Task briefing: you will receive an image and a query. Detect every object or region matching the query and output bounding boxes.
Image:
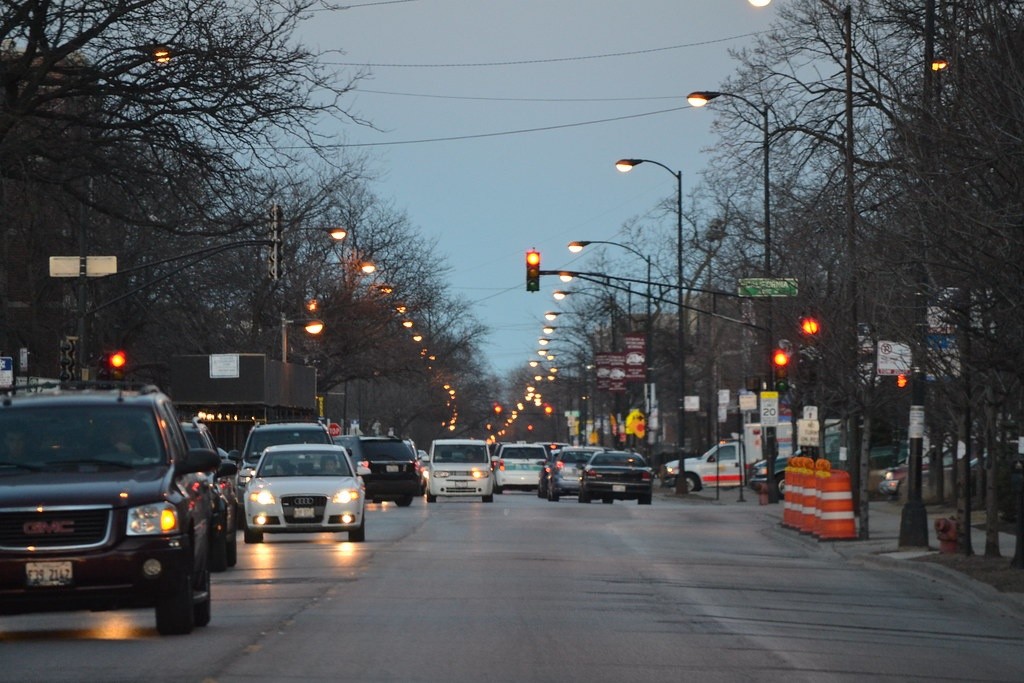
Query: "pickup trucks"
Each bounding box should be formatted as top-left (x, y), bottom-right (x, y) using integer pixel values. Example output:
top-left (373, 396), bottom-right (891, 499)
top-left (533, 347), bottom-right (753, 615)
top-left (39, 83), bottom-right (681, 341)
top-left (749, 432), bottom-right (897, 504)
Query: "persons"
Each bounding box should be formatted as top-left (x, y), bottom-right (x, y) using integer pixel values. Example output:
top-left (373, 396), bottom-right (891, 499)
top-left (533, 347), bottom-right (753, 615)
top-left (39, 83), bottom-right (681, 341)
top-left (324, 457), bottom-right (336, 470)
top-left (466, 449), bottom-right (474, 460)
top-left (109, 418), bottom-right (136, 453)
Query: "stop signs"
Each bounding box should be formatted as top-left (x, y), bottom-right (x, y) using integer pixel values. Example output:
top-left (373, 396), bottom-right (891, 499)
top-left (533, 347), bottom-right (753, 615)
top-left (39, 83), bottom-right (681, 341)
top-left (329, 423), bottom-right (342, 436)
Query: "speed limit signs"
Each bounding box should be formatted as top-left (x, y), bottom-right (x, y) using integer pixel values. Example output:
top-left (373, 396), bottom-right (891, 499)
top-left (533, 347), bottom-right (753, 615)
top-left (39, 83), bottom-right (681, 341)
top-left (759, 392), bottom-right (779, 428)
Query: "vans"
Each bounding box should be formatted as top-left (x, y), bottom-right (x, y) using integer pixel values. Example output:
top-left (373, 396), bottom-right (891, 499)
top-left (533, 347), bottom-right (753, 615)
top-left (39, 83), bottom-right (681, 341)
top-left (491, 444), bottom-right (548, 496)
top-left (421, 438), bottom-right (499, 503)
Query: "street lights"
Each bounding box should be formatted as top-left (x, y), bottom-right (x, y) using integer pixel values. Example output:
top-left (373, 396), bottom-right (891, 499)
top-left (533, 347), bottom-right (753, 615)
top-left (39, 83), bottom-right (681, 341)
top-left (568, 239), bottom-right (652, 468)
top-left (616, 157), bottom-right (688, 495)
top-left (687, 91), bottom-right (776, 507)
top-left (748, 0), bottom-right (860, 523)
top-left (282, 312), bottom-right (324, 363)
top-left (530, 272), bottom-right (637, 450)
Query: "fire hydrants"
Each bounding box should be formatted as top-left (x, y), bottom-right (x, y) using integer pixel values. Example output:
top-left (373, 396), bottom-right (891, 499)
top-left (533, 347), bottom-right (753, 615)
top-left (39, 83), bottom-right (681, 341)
top-left (934, 516), bottom-right (959, 555)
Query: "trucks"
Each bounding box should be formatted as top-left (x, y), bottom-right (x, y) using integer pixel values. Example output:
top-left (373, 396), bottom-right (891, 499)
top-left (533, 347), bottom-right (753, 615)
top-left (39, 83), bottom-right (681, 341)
top-left (660, 416), bottom-right (842, 496)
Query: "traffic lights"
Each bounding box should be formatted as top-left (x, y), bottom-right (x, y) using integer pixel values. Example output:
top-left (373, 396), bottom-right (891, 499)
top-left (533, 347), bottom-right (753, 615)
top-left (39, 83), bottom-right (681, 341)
top-left (526, 252), bottom-right (541, 291)
top-left (773, 353), bottom-right (788, 395)
top-left (108, 351), bottom-right (127, 388)
top-left (896, 374), bottom-right (909, 388)
top-left (96, 356), bottom-right (111, 390)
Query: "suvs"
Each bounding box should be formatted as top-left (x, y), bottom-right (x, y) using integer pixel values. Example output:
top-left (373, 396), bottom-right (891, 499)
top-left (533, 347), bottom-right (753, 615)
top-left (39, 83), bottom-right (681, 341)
top-left (330, 434), bottom-right (425, 507)
top-left (227, 421), bottom-right (353, 530)
top-left (0, 381), bottom-right (223, 637)
top-left (179, 416), bottom-right (240, 572)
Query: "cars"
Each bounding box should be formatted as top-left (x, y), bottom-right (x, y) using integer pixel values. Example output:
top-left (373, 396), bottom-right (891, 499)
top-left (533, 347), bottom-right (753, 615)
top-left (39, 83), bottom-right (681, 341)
top-left (403, 438), bottom-right (432, 482)
top-left (491, 439), bottom-right (571, 456)
top-left (878, 447), bottom-right (989, 506)
top-left (576, 449), bottom-right (654, 505)
top-left (238, 443), bottom-right (373, 544)
top-left (536, 446), bottom-right (605, 502)
